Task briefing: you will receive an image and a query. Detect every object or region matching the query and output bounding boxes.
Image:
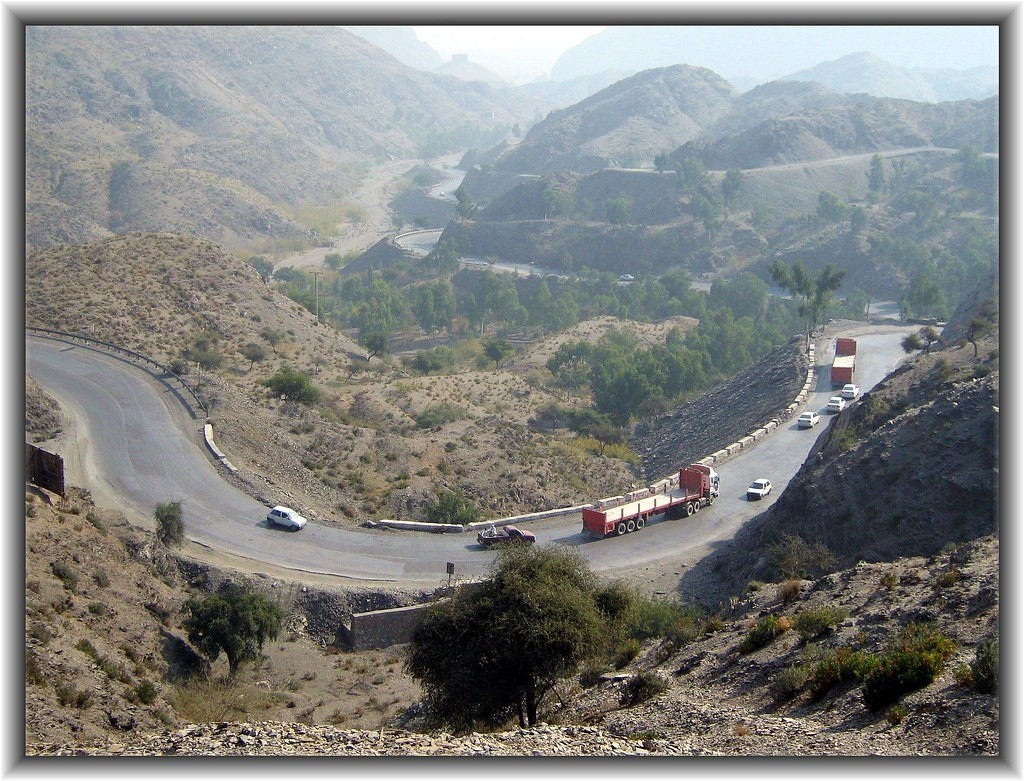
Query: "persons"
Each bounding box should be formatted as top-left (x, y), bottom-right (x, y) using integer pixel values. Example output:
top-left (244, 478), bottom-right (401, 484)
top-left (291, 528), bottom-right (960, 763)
top-left (483, 528), bottom-right (493, 537)
top-left (489, 525), bottom-right (497, 536)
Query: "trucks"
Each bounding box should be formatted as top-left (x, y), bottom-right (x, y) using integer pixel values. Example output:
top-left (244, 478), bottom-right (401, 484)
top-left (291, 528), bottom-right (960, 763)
top-left (829, 337), bottom-right (857, 388)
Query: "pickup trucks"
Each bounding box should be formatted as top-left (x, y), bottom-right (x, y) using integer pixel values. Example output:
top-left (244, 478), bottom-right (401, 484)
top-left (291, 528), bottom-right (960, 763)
top-left (476, 524), bottom-right (536, 551)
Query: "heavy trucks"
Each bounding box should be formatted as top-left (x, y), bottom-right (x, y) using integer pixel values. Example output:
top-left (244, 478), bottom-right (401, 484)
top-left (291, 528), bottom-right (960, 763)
top-left (581, 462), bottom-right (721, 541)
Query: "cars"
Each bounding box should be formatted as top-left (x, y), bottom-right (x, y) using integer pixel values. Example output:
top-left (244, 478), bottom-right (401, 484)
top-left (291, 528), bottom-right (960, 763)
top-left (841, 383), bottom-right (860, 400)
top-left (826, 396), bottom-right (847, 414)
top-left (746, 478), bottom-right (772, 500)
top-left (619, 274), bottom-right (635, 281)
top-left (266, 505), bottom-right (308, 532)
top-left (797, 411), bottom-right (821, 429)
